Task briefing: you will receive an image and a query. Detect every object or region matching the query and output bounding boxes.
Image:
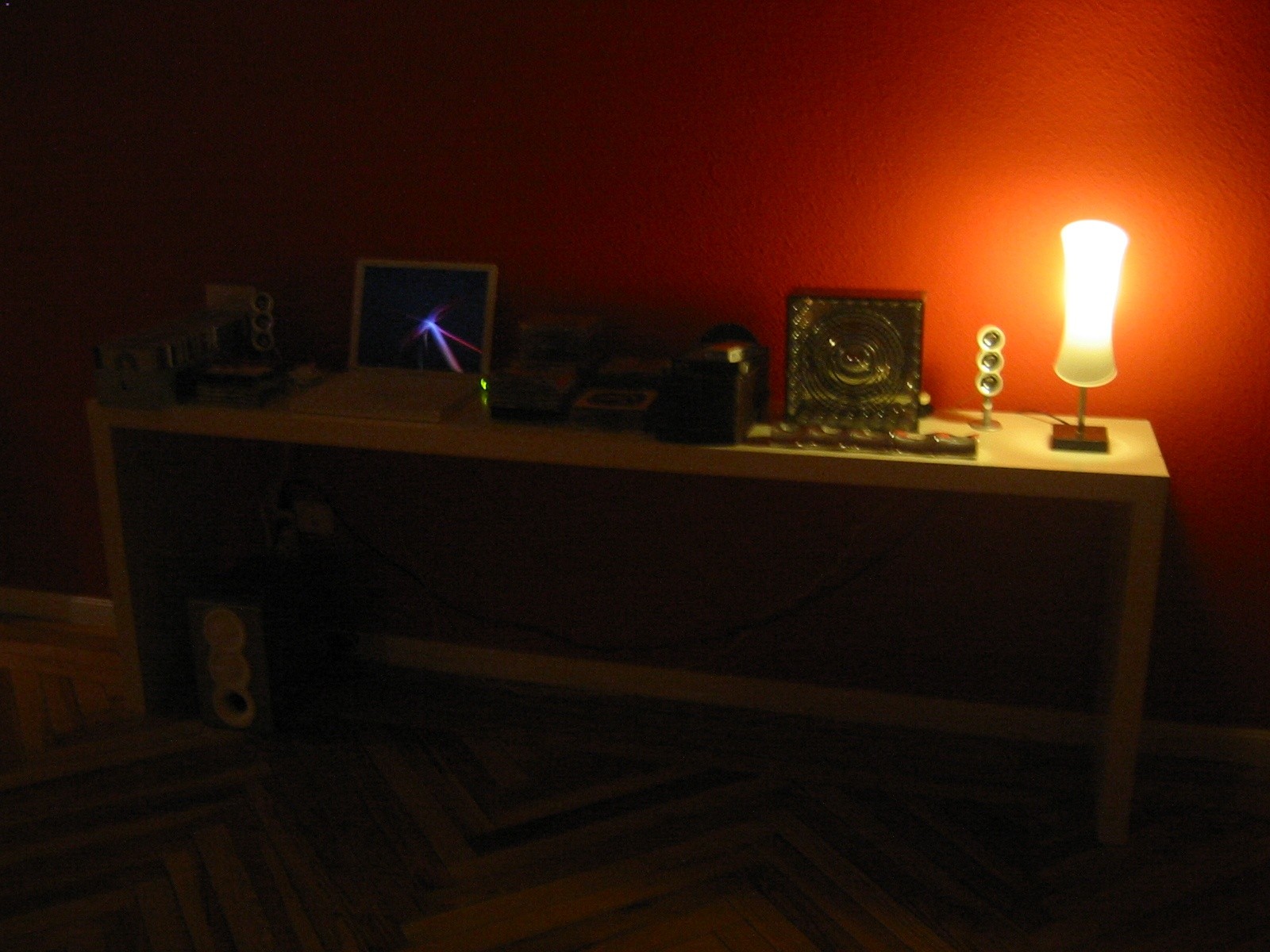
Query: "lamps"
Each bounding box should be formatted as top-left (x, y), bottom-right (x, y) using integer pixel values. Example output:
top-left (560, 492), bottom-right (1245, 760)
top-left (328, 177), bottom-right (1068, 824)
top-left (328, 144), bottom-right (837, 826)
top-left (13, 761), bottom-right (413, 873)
top-left (1051, 219), bottom-right (1128, 452)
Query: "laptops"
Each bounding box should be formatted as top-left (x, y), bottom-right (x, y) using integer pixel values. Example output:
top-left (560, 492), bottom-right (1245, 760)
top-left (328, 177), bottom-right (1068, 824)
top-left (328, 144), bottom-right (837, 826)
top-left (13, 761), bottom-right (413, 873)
top-left (288, 260), bottom-right (497, 424)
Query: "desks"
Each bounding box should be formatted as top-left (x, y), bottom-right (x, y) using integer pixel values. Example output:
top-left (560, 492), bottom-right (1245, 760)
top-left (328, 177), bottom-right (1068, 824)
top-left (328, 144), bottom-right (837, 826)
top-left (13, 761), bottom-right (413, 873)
top-left (84, 358), bottom-right (1170, 842)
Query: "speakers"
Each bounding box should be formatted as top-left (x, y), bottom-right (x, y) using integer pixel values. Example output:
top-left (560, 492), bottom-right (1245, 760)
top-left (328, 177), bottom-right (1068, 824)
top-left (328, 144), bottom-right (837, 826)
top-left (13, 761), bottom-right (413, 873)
top-left (973, 324), bottom-right (1007, 428)
top-left (250, 292), bottom-right (277, 355)
top-left (188, 596), bottom-right (282, 737)
